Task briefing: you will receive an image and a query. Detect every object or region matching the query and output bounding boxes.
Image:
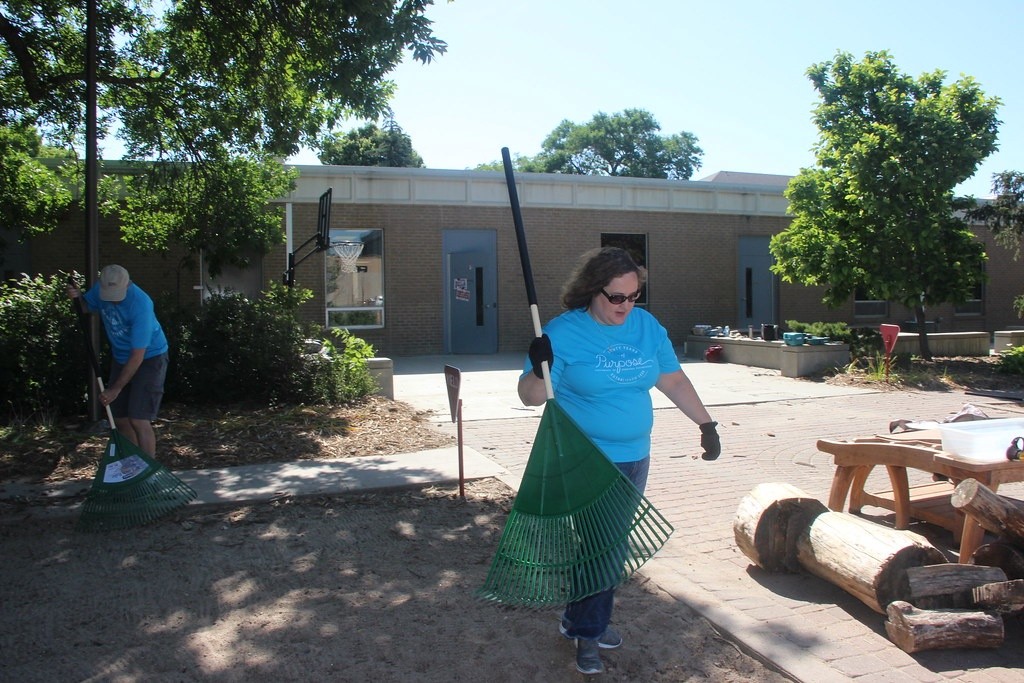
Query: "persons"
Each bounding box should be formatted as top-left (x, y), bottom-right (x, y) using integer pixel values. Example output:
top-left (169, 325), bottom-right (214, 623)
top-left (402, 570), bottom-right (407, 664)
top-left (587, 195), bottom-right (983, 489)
top-left (67, 264), bottom-right (168, 495)
top-left (528, 248), bottom-right (720, 676)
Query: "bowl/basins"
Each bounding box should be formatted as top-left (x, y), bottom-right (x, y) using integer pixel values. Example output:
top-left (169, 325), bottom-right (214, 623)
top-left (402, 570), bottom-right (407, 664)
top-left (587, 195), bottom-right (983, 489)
top-left (691, 328), bottom-right (710, 336)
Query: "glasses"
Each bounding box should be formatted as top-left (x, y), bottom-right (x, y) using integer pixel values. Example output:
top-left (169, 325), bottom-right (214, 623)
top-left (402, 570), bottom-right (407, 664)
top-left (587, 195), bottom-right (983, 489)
top-left (600, 287), bottom-right (641, 305)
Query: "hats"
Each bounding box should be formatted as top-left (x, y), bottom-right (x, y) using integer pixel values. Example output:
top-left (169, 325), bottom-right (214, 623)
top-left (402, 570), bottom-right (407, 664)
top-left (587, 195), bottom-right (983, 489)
top-left (99, 264), bottom-right (129, 301)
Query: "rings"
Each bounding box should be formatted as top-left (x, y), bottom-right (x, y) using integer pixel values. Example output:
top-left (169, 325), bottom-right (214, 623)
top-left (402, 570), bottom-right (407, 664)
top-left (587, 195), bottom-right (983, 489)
top-left (104, 400), bottom-right (107, 402)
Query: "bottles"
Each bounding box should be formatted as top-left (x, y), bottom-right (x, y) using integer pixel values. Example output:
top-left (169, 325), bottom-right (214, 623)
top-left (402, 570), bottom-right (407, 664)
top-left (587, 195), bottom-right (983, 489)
top-left (725, 325), bottom-right (729, 337)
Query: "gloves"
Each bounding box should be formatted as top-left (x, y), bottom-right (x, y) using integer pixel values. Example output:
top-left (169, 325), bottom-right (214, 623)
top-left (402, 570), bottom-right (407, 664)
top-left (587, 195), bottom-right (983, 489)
top-left (699, 422), bottom-right (720, 460)
top-left (528, 334), bottom-right (554, 379)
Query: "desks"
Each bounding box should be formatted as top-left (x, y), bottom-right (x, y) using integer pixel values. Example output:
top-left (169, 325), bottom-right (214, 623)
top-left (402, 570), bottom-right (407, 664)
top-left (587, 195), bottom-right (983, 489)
top-left (817, 415), bottom-right (1024, 565)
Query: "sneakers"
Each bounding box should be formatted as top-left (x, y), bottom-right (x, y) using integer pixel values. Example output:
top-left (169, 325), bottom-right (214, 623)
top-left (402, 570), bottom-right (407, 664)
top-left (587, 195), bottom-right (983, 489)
top-left (559, 620), bottom-right (622, 648)
top-left (572, 637), bottom-right (603, 674)
